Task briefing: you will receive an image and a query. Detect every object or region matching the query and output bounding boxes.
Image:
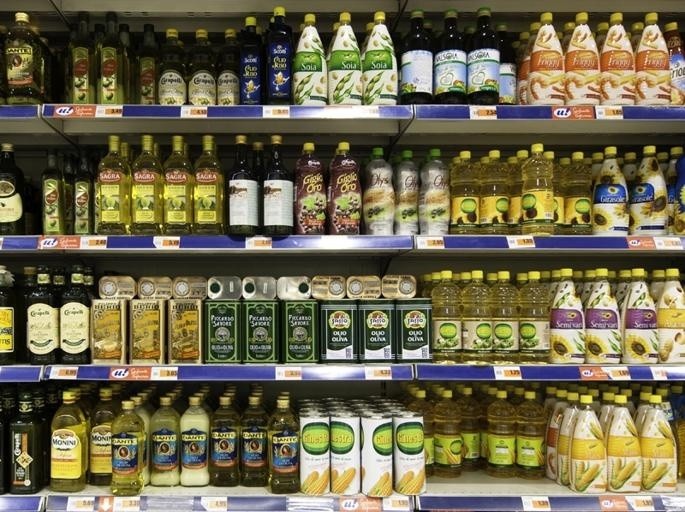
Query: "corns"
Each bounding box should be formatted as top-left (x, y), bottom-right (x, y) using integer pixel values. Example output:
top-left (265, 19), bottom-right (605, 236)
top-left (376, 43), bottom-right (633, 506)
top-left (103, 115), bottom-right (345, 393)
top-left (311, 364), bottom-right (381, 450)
top-left (561, 459), bottom-right (668, 491)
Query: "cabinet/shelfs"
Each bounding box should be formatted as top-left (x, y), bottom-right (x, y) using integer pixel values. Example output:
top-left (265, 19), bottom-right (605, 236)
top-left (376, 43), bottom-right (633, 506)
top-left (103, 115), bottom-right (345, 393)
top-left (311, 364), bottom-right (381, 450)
top-left (0, 0), bottom-right (685, 512)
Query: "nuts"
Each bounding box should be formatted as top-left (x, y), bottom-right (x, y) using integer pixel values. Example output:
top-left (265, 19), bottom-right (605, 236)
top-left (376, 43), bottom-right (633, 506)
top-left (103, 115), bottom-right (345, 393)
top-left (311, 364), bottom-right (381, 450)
top-left (660, 339), bottom-right (675, 361)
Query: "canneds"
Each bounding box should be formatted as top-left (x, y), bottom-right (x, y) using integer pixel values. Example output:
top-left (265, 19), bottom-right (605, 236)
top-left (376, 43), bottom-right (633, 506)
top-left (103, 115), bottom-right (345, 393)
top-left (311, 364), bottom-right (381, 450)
top-left (298, 396), bottom-right (427, 497)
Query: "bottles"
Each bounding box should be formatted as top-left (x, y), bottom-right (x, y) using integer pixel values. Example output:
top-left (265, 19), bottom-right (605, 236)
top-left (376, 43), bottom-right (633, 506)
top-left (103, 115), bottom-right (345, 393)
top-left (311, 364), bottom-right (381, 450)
top-left (0, 383), bottom-right (685, 496)
top-left (0, 261), bottom-right (685, 364)
top-left (3, 8), bottom-right (685, 107)
top-left (4, 133), bottom-right (685, 235)
